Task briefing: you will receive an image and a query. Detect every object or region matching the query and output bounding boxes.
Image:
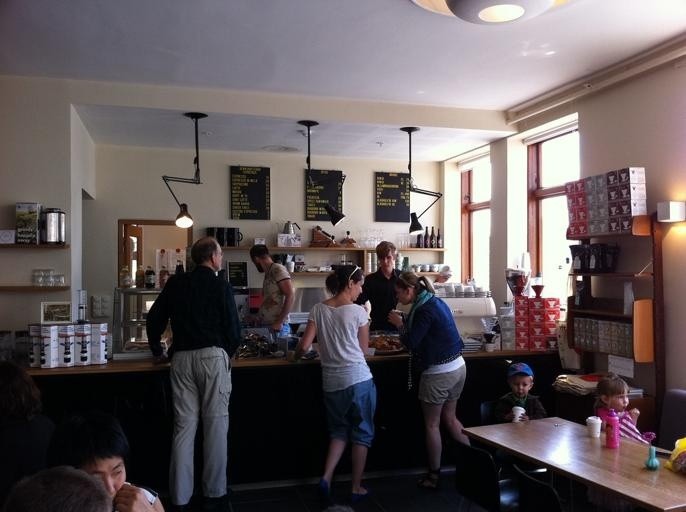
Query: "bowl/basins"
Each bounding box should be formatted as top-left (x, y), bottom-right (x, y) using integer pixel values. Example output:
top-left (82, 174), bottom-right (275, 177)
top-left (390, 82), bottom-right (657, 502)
top-left (410, 263), bottom-right (446, 272)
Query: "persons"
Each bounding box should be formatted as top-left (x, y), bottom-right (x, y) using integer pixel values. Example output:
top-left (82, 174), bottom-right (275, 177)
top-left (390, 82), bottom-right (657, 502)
top-left (389, 270), bottom-right (472, 491)
top-left (355, 240), bottom-right (405, 330)
top-left (5, 462), bottom-right (112, 510)
top-left (47, 412), bottom-right (166, 511)
top-left (146, 236), bottom-right (241, 509)
top-left (592, 374), bottom-right (651, 446)
top-left (243, 245), bottom-right (293, 338)
top-left (294, 263), bottom-right (382, 500)
top-left (495, 363), bottom-right (546, 423)
top-left (1, 359), bottom-right (58, 509)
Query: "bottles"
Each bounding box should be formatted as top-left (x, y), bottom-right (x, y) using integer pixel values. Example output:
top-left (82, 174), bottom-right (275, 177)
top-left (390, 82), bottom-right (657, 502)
top-left (645, 447), bottom-right (660, 471)
top-left (623, 281), bottom-right (634, 316)
top-left (603, 408), bottom-right (621, 449)
top-left (417, 226), bottom-right (443, 248)
top-left (118, 259), bottom-right (184, 289)
top-left (40, 206), bottom-right (66, 244)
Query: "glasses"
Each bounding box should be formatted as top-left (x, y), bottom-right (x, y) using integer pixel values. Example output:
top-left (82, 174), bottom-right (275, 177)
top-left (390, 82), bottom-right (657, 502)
top-left (348, 262), bottom-right (362, 281)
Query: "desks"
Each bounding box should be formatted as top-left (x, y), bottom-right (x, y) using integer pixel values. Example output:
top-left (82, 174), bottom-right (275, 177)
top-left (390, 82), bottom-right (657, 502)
top-left (457, 413), bottom-right (686, 510)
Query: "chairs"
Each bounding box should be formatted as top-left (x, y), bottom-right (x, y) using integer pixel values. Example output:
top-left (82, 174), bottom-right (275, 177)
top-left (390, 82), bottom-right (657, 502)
top-left (451, 440), bottom-right (524, 510)
top-left (513, 459), bottom-right (566, 510)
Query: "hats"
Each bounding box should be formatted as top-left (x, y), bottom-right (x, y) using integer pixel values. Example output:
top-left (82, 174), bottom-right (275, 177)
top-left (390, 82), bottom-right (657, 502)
top-left (506, 362), bottom-right (535, 380)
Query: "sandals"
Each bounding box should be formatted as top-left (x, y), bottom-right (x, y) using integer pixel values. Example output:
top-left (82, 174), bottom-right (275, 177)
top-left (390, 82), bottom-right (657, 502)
top-left (415, 467), bottom-right (442, 492)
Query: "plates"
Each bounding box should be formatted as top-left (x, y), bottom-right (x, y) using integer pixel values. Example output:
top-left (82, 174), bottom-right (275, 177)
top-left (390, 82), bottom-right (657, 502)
top-left (458, 339), bottom-right (481, 353)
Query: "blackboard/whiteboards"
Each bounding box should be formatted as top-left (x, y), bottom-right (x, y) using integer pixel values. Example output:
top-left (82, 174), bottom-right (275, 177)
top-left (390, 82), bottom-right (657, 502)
top-left (230, 166), bottom-right (271, 221)
top-left (226, 260), bottom-right (249, 288)
top-left (374, 172), bottom-right (411, 222)
top-left (305, 168), bottom-right (342, 221)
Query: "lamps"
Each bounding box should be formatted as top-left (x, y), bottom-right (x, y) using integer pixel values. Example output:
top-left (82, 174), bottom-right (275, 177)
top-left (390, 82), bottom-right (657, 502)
top-left (293, 120), bottom-right (346, 228)
top-left (407, 1), bottom-right (578, 31)
top-left (158, 112), bottom-right (210, 230)
top-left (398, 125), bottom-right (444, 237)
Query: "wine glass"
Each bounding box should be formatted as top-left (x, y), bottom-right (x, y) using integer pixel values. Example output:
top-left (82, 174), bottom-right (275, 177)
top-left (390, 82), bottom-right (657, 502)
top-left (531, 284), bottom-right (544, 299)
top-left (567, 171), bottom-right (632, 236)
top-left (518, 300), bottom-right (559, 349)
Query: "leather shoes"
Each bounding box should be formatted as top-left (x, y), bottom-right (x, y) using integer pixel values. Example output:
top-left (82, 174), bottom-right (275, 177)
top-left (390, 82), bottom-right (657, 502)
top-left (173, 487), bottom-right (199, 512)
top-left (347, 483), bottom-right (374, 505)
top-left (200, 486), bottom-right (234, 512)
top-left (318, 477), bottom-right (336, 507)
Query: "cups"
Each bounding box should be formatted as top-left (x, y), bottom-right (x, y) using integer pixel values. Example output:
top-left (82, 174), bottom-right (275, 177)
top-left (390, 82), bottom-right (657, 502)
top-left (288, 262), bottom-right (294, 273)
top-left (512, 406), bottom-right (527, 423)
top-left (29, 267), bottom-right (66, 285)
top-left (505, 267), bottom-right (531, 296)
top-left (500, 306), bottom-right (514, 315)
top-left (366, 251), bottom-right (409, 273)
top-left (392, 310), bottom-right (404, 317)
top-left (482, 317), bottom-right (502, 352)
top-left (433, 283), bottom-right (494, 298)
top-left (586, 415), bottom-right (602, 438)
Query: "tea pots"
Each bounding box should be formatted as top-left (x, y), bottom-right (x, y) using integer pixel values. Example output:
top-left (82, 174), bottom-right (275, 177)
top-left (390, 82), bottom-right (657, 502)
top-left (283, 220), bottom-right (301, 234)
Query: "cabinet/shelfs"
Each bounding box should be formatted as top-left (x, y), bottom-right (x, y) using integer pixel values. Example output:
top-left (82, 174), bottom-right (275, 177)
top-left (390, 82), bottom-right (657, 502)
top-left (0, 240), bottom-right (70, 292)
top-left (557, 206), bottom-right (667, 438)
top-left (192, 241), bottom-right (448, 283)
